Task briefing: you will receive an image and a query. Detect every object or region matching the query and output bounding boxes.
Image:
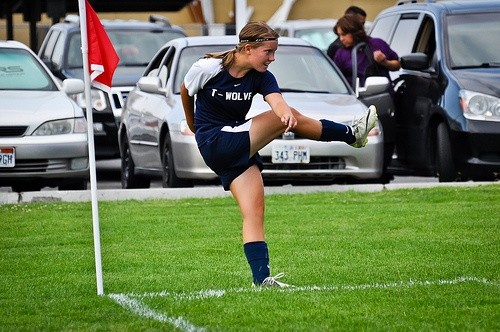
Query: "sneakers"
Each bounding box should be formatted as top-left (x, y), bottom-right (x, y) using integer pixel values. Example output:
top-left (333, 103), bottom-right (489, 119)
top-left (252, 277), bottom-right (289, 288)
top-left (350, 104), bottom-right (378, 148)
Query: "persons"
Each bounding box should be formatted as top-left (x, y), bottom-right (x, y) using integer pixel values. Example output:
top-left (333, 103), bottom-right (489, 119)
top-left (181, 21), bottom-right (379, 288)
top-left (328, 6), bottom-right (400, 185)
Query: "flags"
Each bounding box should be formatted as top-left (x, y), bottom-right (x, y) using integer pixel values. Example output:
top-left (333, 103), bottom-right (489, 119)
top-left (79, 0), bottom-right (120, 93)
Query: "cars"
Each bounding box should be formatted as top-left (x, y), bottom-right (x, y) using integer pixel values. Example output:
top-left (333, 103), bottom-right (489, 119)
top-left (118, 35), bottom-right (385, 189)
top-left (0, 40), bottom-right (90, 192)
top-left (272, 20), bottom-right (374, 55)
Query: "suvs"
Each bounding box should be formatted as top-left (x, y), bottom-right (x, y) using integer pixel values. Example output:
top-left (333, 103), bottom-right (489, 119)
top-left (37, 14), bottom-right (188, 136)
top-left (369, 0), bottom-right (500, 180)
